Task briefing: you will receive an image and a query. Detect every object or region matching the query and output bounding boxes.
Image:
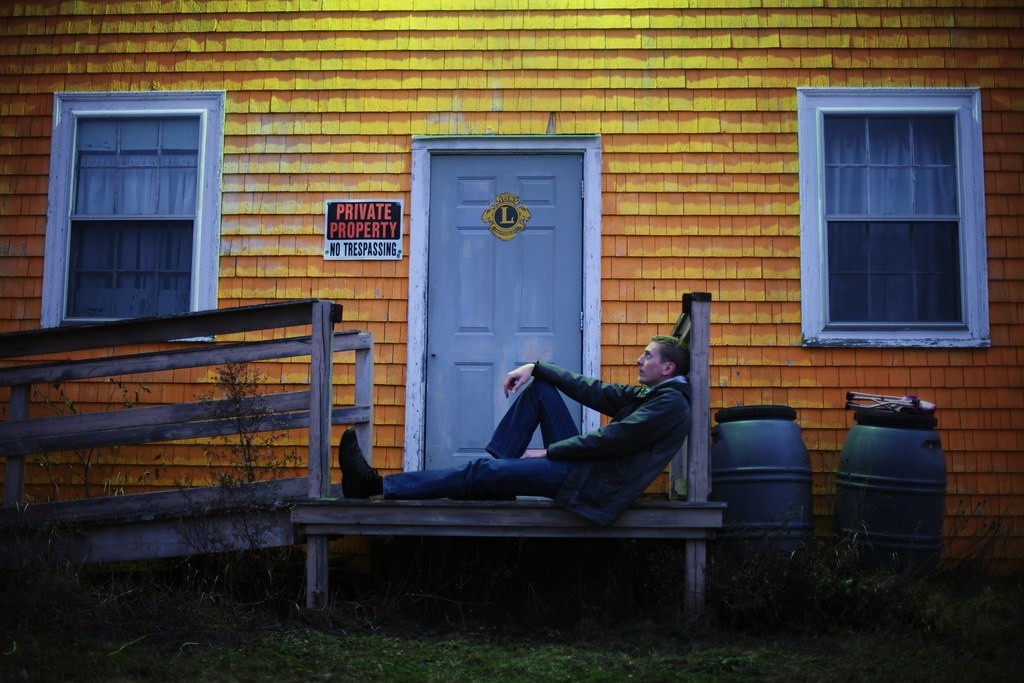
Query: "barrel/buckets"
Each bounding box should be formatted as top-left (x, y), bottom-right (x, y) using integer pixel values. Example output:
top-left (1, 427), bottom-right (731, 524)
top-left (702, 418), bottom-right (816, 602)
top-left (831, 424), bottom-right (947, 581)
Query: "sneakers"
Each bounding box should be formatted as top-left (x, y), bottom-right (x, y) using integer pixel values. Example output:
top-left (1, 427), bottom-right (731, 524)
top-left (339, 427), bottom-right (379, 499)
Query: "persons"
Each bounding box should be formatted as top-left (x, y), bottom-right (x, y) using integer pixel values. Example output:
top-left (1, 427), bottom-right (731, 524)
top-left (336, 334), bottom-right (689, 527)
